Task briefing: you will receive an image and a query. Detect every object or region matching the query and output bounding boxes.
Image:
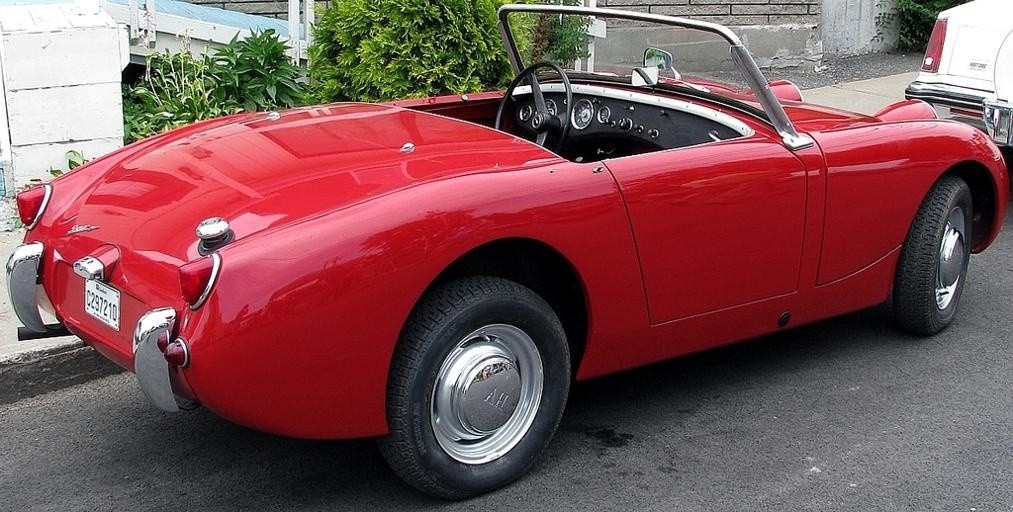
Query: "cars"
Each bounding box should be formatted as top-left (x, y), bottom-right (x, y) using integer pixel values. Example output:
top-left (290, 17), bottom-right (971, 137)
top-left (903, 2), bottom-right (1013, 157)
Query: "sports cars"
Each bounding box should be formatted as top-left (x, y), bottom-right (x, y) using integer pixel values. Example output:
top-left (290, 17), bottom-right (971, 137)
top-left (5, 5), bottom-right (1008, 502)
